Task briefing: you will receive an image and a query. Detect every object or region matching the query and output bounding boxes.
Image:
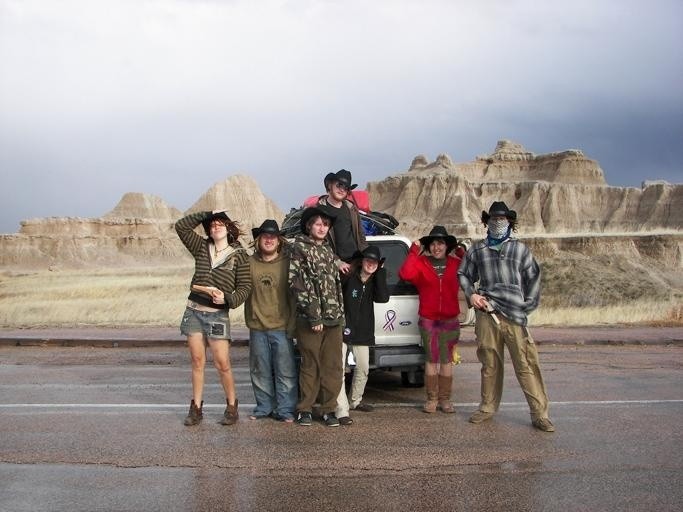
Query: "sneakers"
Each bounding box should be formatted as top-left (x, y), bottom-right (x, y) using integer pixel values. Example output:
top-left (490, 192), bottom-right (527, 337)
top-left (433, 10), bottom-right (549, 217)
top-left (532, 417), bottom-right (555, 432)
top-left (338, 417), bottom-right (353, 426)
top-left (297, 412), bottom-right (313, 426)
top-left (320, 411), bottom-right (340, 427)
top-left (350, 403), bottom-right (375, 412)
top-left (469, 409), bottom-right (495, 424)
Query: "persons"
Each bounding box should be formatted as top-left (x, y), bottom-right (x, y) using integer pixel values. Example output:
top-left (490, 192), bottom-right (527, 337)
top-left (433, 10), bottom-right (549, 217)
top-left (398, 225), bottom-right (468, 413)
top-left (175, 209), bottom-right (253, 427)
top-left (456, 200), bottom-right (556, 432)
top-left (304, 168), bottom-right (370, 281)
top-left (288, 206), bottom-right (346, 427)
top-left (245, 219), bottom-right (303, 423)
top-left (335, 245), bottom-right (389, 425)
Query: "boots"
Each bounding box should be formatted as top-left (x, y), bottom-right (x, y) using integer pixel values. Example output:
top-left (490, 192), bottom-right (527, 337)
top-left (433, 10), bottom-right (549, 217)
top-left (220, 397), bottom-right (240, 426)
top-left (423, 373), bottom-right (456, 414)
top-left (183, 399), bottom-right (204, 427)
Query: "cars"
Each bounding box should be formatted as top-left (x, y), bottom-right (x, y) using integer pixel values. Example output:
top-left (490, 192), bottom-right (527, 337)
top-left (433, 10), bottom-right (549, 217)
top-left (281, 234), bottom-right (473, 389)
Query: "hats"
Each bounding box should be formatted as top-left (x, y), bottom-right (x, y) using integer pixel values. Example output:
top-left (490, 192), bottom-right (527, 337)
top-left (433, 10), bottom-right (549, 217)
top-left (251, 219), bottom-right (287, 241)
top-left (300, 206), bottom-right (338, 237)
top-left (202, 212), bottom-right (239, 243)
top-left (419, 225), bottom-right (458, 256)
top-left (352, 245), bottom-right (386, 271)
top-left (324, 169), bottom-right (358, 191)
top-left (481, 200), bottom-right (517, 224)
